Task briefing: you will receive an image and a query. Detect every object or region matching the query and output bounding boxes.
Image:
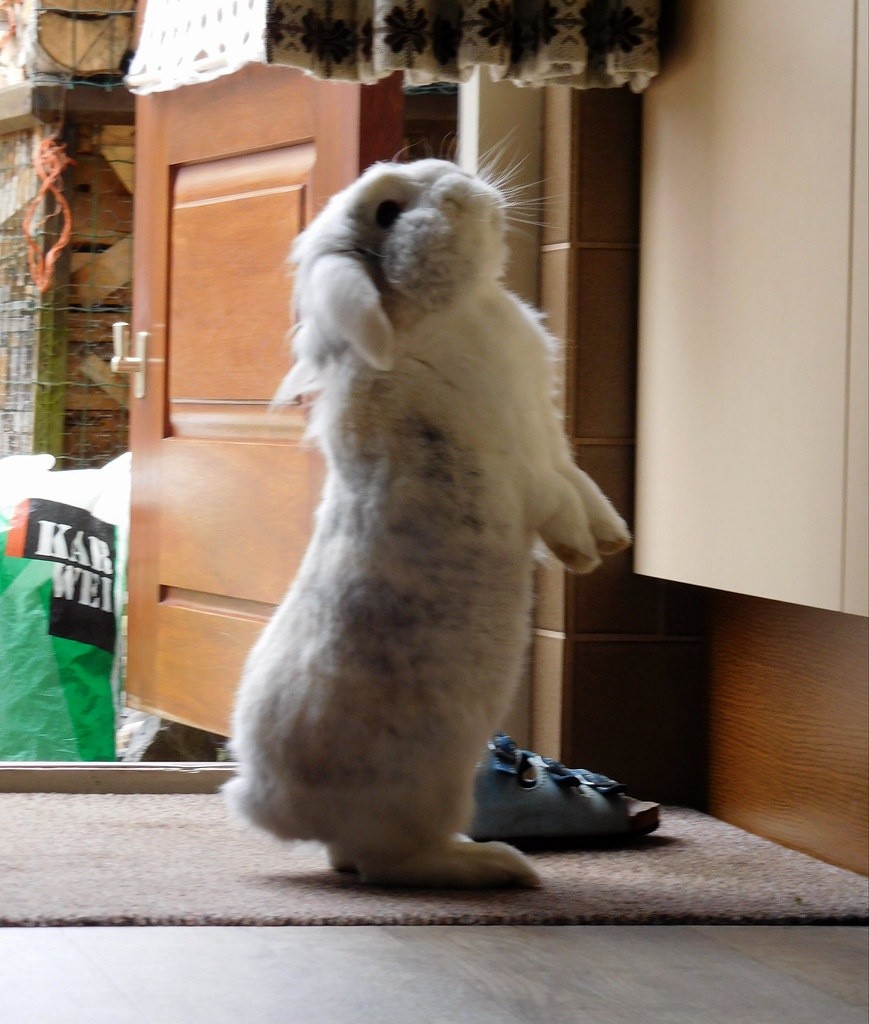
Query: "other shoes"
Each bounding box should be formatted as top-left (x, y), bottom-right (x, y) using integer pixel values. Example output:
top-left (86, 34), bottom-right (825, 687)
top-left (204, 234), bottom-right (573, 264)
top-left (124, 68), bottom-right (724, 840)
top-left (463, 733), bottom-right (662, 847)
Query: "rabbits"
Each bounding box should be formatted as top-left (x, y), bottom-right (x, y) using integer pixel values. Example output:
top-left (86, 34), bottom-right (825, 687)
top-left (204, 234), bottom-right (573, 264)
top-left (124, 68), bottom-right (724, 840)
top-left (212, 128), bottom-right (630, 888)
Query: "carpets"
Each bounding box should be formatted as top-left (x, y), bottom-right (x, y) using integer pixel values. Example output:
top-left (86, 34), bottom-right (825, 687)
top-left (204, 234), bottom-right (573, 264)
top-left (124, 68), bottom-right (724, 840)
top-left (0, 791), bottom-right (869, 929)
